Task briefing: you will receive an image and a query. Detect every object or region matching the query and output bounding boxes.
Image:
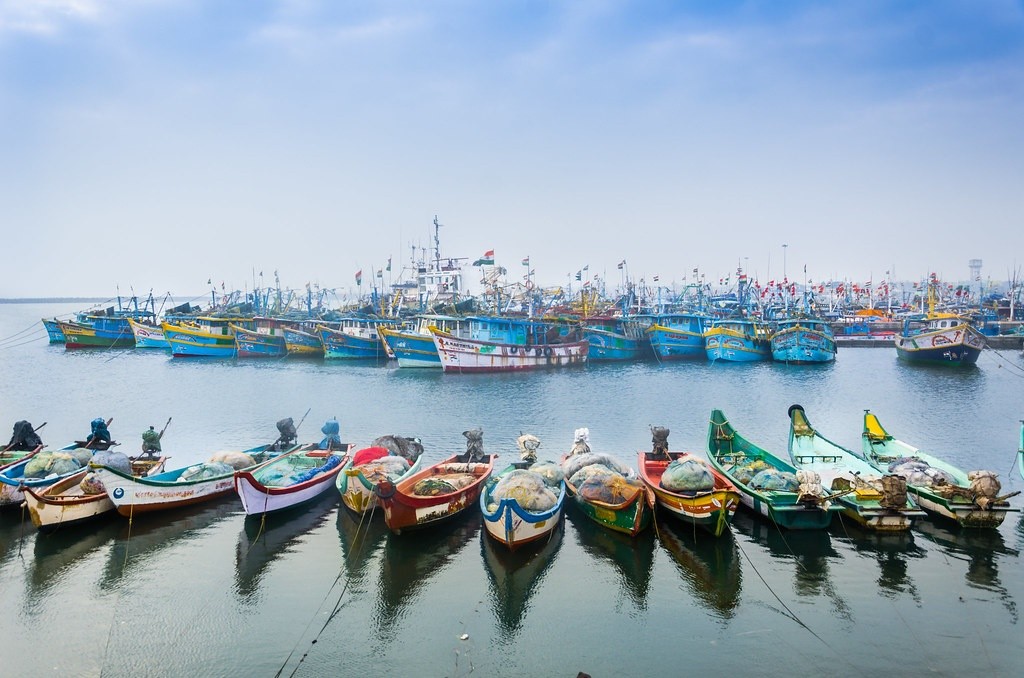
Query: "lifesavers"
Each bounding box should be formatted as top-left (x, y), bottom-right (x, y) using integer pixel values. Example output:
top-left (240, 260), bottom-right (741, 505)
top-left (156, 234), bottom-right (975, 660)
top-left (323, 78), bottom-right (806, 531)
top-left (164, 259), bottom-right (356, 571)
top-left (536, 349), bottom-right (542, 355)
top-left (753, 338), bottom-right (759, 346)
top-left (760, 339), bottom-right (769, 345)
top-left (544, 347), bottom-right (552, 356)
top-left (511, 346), bottom-right (517, 353)
top-left (951, 308), bottom-right (959, 315)
top-left (525, 347), bottom-right (531, 351)
top-left (637, 339), bottom-right (641, 346)
top-left (375, 481), bottom-right (396, 498)
top-left (745, 335), bottom-right (750, 340)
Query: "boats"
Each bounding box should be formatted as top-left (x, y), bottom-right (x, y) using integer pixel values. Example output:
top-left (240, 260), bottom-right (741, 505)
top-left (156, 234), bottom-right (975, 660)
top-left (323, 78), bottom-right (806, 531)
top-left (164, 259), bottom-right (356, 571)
top-left (336, 434), bottom-right (425, 515)
top-left (0, 416), bottom-right (169, 530)
top-left (43, 212), bottom-right (1024, 373)
top-left (637, 425), bottom-right (742, 528)
top-left (87, 416), bottom-right (303, 522)
top-left (1018, 422), bottom-right (1024, 478)
top-left (376, 428), bottom-right (499, 533)
top-left (706, 408), bottom-right (848, 531)
top-left (787, 404), bottom-right (928, 531)
top-left (562, 428), bottom-right (646, 536)
top-left (862, 409), bottom-right (1011, 527)
top-left (480, 433), bottom-right (566, 550)
top-left (234, 419), bottom-right (355, 517)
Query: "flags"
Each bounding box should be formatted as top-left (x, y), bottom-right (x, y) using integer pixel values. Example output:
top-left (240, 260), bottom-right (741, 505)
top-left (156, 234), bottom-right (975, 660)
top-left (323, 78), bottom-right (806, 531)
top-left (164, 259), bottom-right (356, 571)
top-left (693, 268), bottom-right (705, 279)
top-left (653, 275), bottom-right (658, 281)
top-left (472, 250), bottom-right (495, 266)
top-left (804, 265), bottom-right (904, 306)
top-left (912, 273), bottom-right (969, 299)
top-left (975, 275), bottom-right (998, 294)
top-left (761, 277), bottom-right (795, 301)
top-left (306, 282), bottom-right (319, 292)
top-left (442, 276), bottom-right (454, 289)
top-left (355, 271), bottom-right (362, 286)
top-left (682, 276), bottom-right (687, 279)
top-left (258, 270), bottom-right (280, 282)
top-left (719, 267), bottom-right (760, 289)
top-left (208, 279), bottom-right (226, 292)
top-left (117, 285), bottom-right (154, 292)
top-left (522, 259), bottom-right (535, 280)
top-left (377, 258), bottom-right (391, 277)
top-left (617, 259), bottom-right (626, 270)
top-left (640, 278), bottom-right (644, 283)
top-left (566, 264), bottom-right (598, 288)
